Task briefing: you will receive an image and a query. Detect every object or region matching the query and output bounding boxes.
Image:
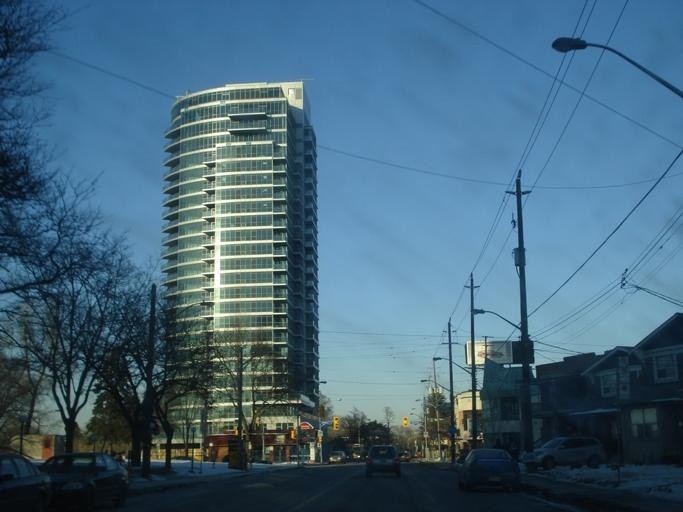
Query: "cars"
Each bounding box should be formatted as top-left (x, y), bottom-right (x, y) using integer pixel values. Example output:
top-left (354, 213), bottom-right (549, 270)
top-left (0, 453), bottom-right (51, 511)
top-left (38, 452), bottom-right (128, 511)
top-left (363, 444), bottom-right (402, 478)
top-left (327, 443), bottom-right (411, 465)
top-left (457, 447), bottom-right (521, 492)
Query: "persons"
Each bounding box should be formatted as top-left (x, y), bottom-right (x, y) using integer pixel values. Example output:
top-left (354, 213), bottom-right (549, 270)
top-left (460, 444), bottom-right (469, 459)
top-left (493, 438), bottom-right (505, 449)
top-left (346, 455), bottom-right (350, 462)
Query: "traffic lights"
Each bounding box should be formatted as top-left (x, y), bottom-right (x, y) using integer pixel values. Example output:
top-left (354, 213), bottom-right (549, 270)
top-left (402, 415), bottom-right (408, 426)
top-left (333, 416), bottom-right (339, 431)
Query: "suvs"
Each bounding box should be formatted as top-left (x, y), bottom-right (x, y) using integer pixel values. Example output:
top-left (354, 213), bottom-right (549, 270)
top-left (521, 435), bottom-right (606, 471)
top-left (538, 434), bottom-right (613, 459)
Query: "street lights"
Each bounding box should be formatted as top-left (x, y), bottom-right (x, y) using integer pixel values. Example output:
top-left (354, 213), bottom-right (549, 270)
top-left (408, 398), bottom-right (442, 460)
top-left (296, 380), bottom-right (327, 464)
top-left (189, 425), bottom-right (196, 471)
top-left (18, 413), bottom-right (28, 457)
top-left (420, 379), bottom-right (455, 464)
top-left (140, 300), bottom-right (214, 478)
top-left (551, 37), bottom-right (682, 99)
top-left (432, 357), bottom-right (476, 450)
top-left (319, 398), bottom-right (341, 463)
top-left (470, 308), bottom-right (535, 474)
